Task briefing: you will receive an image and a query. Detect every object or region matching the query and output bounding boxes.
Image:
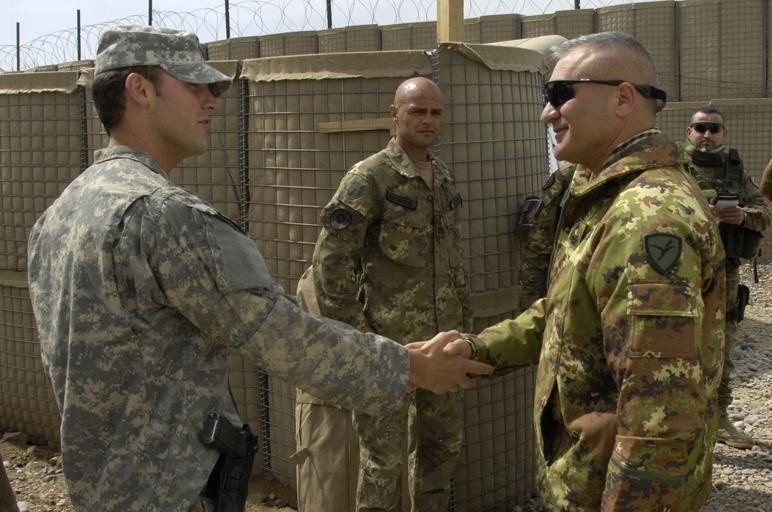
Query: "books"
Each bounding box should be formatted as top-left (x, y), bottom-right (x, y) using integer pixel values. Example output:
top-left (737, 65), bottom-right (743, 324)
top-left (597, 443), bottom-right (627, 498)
top-left (712, 193), bottom-right (741, 223)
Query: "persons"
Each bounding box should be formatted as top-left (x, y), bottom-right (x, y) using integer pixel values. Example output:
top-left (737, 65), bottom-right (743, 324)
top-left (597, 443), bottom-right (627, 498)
top-left (673, 106), bottom-right (772, 450)
top-left (310, 76), bottom-right (478, 511)
top-left (25, 25), bottom-right (496, 512)
top-left (401, 31), bottom-right (727, 512)
top-left (520, 161), bottom-right (577, 312)
top-left (759, 158), bottom-right (772, 201)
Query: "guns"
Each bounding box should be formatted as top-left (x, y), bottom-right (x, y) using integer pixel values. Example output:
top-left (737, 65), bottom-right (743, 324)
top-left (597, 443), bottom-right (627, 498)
top-left (195, 413), bottom-right (258, 511)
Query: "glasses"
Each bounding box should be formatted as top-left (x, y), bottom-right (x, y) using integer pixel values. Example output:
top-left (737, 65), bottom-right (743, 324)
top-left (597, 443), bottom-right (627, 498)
top-left (688, 120), bottom-right (727, 135)
top-left (541, 75), bottom-right (642, 109)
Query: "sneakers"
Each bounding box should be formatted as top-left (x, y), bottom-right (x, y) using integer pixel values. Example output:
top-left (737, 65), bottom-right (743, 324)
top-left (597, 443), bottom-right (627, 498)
top-left (715, 405), bottom-right (754, 449)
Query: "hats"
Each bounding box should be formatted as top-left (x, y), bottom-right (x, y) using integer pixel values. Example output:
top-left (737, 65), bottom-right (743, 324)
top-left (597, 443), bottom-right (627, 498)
top-left (93, 25), bottom-right (233, 99)
